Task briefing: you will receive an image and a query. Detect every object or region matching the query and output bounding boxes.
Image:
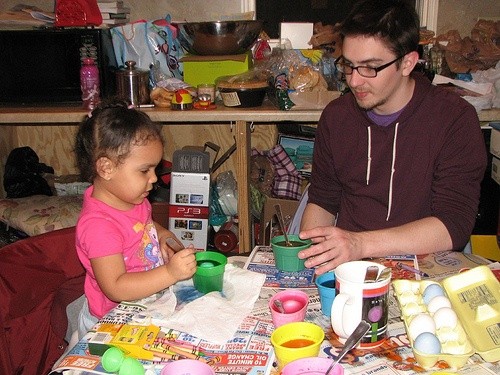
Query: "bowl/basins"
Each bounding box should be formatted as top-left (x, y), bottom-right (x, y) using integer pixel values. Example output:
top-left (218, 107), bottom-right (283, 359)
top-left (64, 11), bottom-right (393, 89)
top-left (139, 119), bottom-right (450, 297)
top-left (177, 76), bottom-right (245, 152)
top-left (170, 20), bottom-right (263, 56)
top-left (217, 86), bottom-right (270, 108)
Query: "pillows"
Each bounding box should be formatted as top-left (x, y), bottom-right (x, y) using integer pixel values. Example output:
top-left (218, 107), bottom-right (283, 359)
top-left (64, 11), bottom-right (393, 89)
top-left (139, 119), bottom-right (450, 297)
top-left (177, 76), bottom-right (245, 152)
top-left (0, 195), bottom-right (80, 237)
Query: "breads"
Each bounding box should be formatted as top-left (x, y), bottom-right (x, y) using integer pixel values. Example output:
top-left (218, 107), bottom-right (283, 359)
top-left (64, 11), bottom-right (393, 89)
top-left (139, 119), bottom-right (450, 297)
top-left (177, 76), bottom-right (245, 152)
top-left (289, 69), bottom-right (328, 90)
top-left (149, 85), bottom-right (176, 108)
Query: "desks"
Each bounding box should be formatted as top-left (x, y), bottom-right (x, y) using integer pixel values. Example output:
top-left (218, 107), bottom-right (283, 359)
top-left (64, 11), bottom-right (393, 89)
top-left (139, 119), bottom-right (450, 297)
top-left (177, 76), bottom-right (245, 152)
top-left (48, 251), bottom-right (492, 374)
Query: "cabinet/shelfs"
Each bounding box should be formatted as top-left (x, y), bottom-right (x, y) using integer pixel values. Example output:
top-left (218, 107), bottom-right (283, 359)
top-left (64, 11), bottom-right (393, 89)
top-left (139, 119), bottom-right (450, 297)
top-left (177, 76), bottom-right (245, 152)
top-left (0, 107), bottom-right (500, 255)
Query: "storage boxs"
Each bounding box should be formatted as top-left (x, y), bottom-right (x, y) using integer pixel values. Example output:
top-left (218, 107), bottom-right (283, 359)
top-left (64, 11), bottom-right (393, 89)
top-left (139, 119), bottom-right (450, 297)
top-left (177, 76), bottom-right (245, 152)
top-left (0, 29), bottom-right (117, 107)
top-left (183, 50), bottom-right (253, 87)
top-left (261, 196), bottom-right (298, 246)
top-left (274, 123), bottom-right (316, 176)
top-left (167, 146), bottom-right (211, 254)
top-left (267, 84), bottom-right (341, 110)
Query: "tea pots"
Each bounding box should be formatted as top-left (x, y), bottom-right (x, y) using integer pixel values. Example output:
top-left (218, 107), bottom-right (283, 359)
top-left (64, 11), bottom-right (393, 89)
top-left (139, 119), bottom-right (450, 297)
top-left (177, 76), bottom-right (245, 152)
top-left (170, 88), bottom-right (192, 111)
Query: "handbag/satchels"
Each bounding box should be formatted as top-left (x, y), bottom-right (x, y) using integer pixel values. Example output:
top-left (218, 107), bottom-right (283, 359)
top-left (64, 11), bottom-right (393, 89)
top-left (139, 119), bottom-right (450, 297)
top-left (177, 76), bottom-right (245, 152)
top-left (5, 147), bottom-right (55, 200)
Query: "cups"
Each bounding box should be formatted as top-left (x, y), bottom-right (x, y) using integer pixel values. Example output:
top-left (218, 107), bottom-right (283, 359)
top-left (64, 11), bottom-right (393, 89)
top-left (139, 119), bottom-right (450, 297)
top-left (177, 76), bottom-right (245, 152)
top-left (269, 235), bottom-right (312, 272)
top-left (192, 251), bottom-right (227, 294)
top-left (270, 321), bottom-right (325, 368)
top-left (330, 261), bottom-right (391, 349)
top-left (269, 289), bottom-right (309, 329)
top-left (315, 272), bottom-right (335, 317)
top-left (279, 357), bottom-right (344, 375)
top-left (159, 359), bottom-right (216, 375)
top-left (197, 84), bottom-right (215, 104)
top-left (113, 61), bottom-right (150, 106)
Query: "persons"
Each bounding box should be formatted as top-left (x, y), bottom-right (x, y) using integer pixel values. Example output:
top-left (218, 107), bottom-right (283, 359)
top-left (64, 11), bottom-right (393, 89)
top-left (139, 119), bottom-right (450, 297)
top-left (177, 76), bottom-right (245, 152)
top-left (297, 0), bottom-right (489, 275)
top-left (76, 102), bottom-right (197, 341)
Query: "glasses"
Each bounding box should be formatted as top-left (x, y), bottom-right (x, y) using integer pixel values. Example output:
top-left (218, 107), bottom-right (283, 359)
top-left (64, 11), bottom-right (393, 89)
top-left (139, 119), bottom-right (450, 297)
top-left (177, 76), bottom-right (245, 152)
top-left (334, 54), bottom-right (404, 78)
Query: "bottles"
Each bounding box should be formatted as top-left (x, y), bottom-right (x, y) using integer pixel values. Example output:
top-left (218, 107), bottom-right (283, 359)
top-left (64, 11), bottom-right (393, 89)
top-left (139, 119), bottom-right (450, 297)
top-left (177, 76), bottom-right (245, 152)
top-left (213, 216), bottom-right (239, 252)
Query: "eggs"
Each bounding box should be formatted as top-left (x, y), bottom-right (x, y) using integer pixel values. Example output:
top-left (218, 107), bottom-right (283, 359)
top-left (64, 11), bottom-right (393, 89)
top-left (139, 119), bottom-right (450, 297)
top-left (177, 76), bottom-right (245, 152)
top-left (200, 262), bottom-right (213, 268)
top-left (408, 313), bottom-right (441, 354)
top-left (282, 300), bottom-right (301, 313)
top-left (423, 283), bottom-right (457, 329)
top-left (102, 347), bottom-right (145, 375)
